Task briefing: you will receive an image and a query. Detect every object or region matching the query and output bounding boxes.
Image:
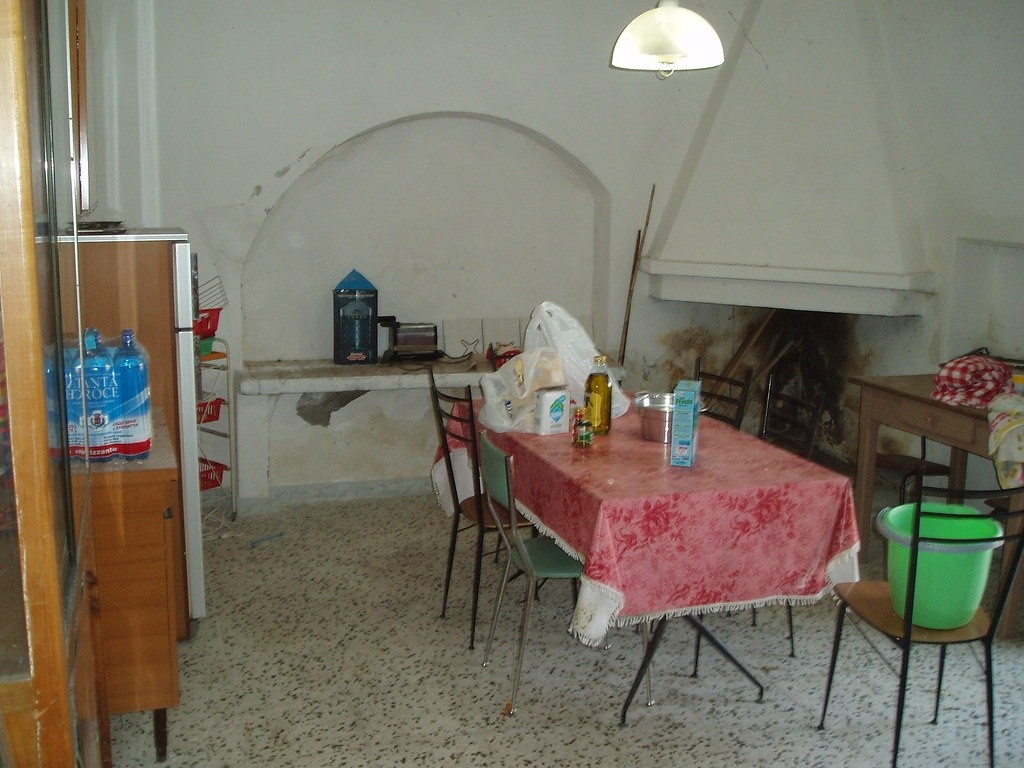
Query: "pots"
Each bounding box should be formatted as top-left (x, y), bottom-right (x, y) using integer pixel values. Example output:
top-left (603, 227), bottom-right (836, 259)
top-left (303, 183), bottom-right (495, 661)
top-left (634, 390), bottom-right (709, 444)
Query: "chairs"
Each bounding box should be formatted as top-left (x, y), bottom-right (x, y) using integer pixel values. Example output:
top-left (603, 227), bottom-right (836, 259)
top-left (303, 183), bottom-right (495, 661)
top-left (478, 429), bottom-right (652, 719)
top-left (429, 383), bottom-right (579, 651)
top-left (817, 437), bottom-right (1024, 768)
top-left (694, 358), bottom-right (755, 429)
top-left (688, 373), bottom-right (829, 678)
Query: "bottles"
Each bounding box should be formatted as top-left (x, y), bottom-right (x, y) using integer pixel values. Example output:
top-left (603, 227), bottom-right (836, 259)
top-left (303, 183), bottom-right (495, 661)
top-left (572, 407), bottom-right (594, 448)
top-left (584, 355), bottom-right (611, 434)
top-left (83, 327), bottom-right (151, 461)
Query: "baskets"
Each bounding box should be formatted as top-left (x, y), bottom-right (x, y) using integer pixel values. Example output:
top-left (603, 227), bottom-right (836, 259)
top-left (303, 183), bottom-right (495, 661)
top-left (199, 457), bottom-right (227, 491)
top-left (193, 307), bottom-right (222, 357)
top-left (196, 392), bottom-right (224, 424)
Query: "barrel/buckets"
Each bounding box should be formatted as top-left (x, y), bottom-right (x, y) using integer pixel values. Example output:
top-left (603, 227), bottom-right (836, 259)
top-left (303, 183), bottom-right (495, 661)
top-left (875, 501), bottom-right (1003, 628)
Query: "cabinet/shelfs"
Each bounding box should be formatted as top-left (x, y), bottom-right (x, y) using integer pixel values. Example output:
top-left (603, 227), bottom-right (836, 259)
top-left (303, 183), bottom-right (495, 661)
top-left (194, 337), bottom-right (237, 522)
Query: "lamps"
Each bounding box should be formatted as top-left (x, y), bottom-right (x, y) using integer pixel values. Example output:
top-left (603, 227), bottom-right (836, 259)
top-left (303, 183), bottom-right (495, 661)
top-left (610, 0), bottom-right (726, 78)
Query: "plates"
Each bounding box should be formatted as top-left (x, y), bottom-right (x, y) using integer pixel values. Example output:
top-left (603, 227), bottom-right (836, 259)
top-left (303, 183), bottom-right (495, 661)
top-left (68, 222), bottom-right (122, 230)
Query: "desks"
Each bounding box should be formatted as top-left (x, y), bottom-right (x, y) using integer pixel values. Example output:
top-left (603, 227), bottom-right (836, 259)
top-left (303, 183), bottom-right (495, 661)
top-left (91, 406), bottom-right (180, 762)
top-left (239, 351), bottom-right (624, 516)
top-left (845, 373), bottom-right (1024, 639)
top-left (453, 388), bottom-right (852, 727)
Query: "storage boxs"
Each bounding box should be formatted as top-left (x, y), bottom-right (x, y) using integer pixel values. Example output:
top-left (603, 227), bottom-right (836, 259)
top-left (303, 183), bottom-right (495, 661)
top-left (200, 338), bottom-right (213, 356)
top-left (193, 307), bottom-right (223, 336)
top-left (670, 380), bottom-right (703, 467)
top-left (533, 384), bottom-right (571, 437)
top-left (199, 457), bottom-right (227, 490)
top-left (196, 397), bottom-right (225, 424)
